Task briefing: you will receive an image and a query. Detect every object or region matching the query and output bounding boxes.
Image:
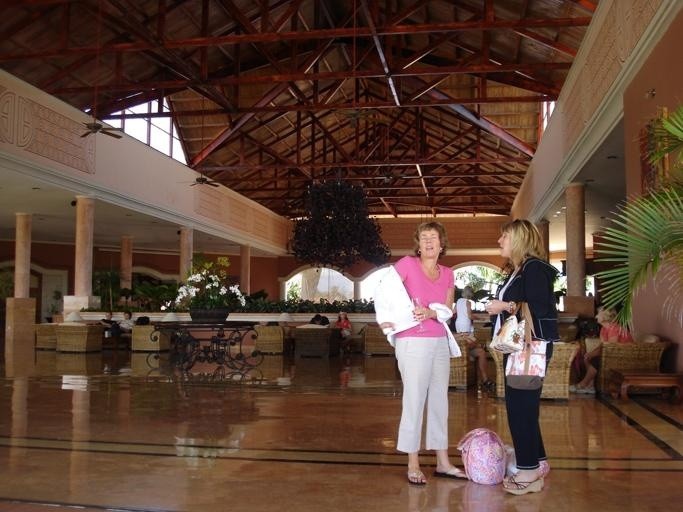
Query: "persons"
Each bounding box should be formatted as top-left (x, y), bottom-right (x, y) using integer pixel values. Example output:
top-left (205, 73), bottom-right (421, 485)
top-left (308, 314), bottom-right (330, 326)
top-left (451, 296), bottom-right (496, 390)
top-left (380, 220), bottom-right (469, 485)
top-left (484, 218), bottom-right (559, 496)
top-left (94, 311), bottom-right (116, 335)
top-left (118, 311), bottom-right (135, 334)
top-left (335, 311), bottom-right (353, 354)
top-left (568, 306), bottom-right (634, 394)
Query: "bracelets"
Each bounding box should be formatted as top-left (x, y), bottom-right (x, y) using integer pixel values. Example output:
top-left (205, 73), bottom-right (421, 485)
top-left (509, 301), bottom-right (515, 314)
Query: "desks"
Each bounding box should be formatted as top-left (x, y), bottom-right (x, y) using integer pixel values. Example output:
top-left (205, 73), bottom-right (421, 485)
top-left (608, 367), bottom-right (682, 400)
top-left (148, 318), bottom-right (261, 368)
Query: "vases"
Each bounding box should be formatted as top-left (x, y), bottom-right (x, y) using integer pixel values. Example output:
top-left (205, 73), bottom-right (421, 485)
top-left (186, 307), bottom-right (232, 321)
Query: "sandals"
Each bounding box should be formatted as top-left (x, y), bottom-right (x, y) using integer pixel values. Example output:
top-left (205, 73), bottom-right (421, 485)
top-left (478, 343), bottom-right (489, 352)
top-left (570, 382), bottom-right (581, 393)
top-left (480, 379), bottom-right (496, 386)
top-left (577, 385), bottom-right (596, 394)
top-left (455, 334), bottom-right (479, 350)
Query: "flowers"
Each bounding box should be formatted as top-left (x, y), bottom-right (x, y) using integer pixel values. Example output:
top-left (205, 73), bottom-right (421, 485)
top-left (159, 253), bottom-right (248, 311)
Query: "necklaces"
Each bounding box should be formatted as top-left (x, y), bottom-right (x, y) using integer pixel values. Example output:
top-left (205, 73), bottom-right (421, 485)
top-left (423, 265), bottom-right (437, 279)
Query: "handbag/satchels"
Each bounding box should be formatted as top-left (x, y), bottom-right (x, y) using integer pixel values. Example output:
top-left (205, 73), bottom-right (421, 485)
top-left (488, 305), bottom-right (525, 354)
top-left (503, 301), bottom-right (547, 391)
top-left (574, 319), bottom-right (600, 340)
top-left (456, 426), bottom-right (507, 486)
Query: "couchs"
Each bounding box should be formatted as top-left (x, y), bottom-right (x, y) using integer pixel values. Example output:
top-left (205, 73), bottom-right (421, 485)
top-left (252, 324), bottom-right (293, 354)
top-left (290, 325), bottom-right (344, 361)
top-left (362, 323), bottom-right (396, 357)
top-left (487, 339), bottom-right (579, 399)
top-left (31, 323), bottom-right (57, 349)
top-left (131, 323), bottom-right (174, 352)
top-left (446, 341), bottom-right (477, 390)
top-left (52, 324), bottom-right (104, 352)
top-left (584, 334), bottom-right (669, 394)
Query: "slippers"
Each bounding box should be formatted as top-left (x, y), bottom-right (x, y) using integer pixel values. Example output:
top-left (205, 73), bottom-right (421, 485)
top-left (503, 471), bottom-right (545, 490)
top-left (433, 466), bottom-right (468, 480)
top-left (501, 478), bottom-right (542, 495)
top-left (407, 468), bottom-right (428, 485)
